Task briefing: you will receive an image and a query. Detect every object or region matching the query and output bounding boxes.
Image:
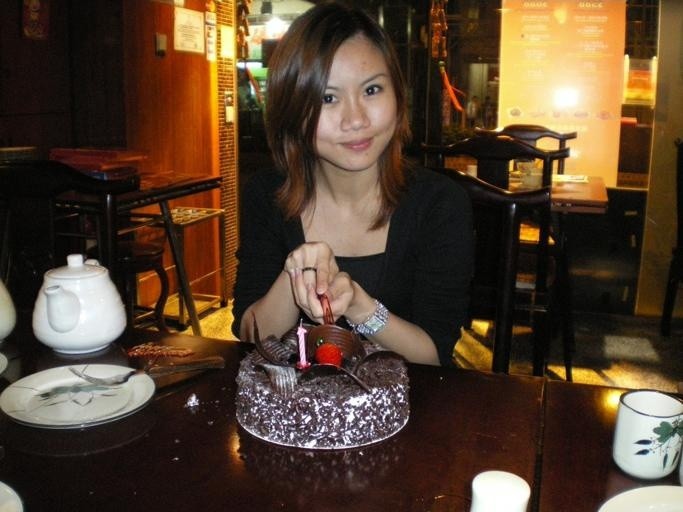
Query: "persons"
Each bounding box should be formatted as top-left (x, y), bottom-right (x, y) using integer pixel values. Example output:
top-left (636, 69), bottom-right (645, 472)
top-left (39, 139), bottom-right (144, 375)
top-left (465, 96), bottom-right (478, 127)
top-left (482, 95), bottom-right (492, 129)
top-left (228, 1), bottom-right (479, 368)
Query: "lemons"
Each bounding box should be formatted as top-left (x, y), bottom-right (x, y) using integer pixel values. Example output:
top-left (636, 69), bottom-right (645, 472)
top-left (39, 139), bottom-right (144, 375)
top-left (29, 252), bottom-right (129, 356)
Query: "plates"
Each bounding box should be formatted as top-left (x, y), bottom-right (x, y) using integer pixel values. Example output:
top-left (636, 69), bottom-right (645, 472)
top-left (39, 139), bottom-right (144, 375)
top-left (151, 205), bottom-right (229, 331)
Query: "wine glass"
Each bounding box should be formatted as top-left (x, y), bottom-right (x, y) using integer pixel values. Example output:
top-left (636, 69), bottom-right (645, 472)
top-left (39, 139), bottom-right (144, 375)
top-left (301, 266), bottom-right (316, 274)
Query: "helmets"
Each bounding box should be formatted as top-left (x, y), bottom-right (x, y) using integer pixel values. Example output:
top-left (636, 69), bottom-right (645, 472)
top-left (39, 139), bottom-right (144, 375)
top-left (1, 362), bottom-right (157, 430)
top-left (596, 484), bottom-right (682, 512)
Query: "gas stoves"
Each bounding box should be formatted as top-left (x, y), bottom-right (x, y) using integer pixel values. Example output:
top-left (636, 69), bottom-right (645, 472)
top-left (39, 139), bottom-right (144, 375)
top-left (235, 311), bottom-right (411, 448)
top-left (237, 423), bottom-right (408, 493)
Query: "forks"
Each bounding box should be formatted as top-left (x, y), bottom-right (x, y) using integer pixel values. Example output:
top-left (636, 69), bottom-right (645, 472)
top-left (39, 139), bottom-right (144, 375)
top-left (66, 360), bottom-right (224, 386)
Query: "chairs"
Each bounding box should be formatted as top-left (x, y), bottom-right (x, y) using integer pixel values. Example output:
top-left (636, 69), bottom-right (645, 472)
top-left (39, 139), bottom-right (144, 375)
top-left (408, 124), bottom-right (577, 375)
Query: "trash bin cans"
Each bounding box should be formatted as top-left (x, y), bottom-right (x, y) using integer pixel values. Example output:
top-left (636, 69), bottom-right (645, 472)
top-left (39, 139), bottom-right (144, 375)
top-left (345, 297), bottom-right (390, 335)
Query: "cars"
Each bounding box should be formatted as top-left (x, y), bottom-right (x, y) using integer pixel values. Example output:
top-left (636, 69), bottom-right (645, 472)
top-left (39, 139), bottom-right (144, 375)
top-left (466, 467), bottom-right (532, 510)
top-left (610, 389), bottom-right (683, 482)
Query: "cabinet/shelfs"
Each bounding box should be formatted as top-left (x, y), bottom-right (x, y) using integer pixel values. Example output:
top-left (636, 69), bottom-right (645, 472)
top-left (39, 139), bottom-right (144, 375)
top-left (151, 205), bottom-right (229, 331)
